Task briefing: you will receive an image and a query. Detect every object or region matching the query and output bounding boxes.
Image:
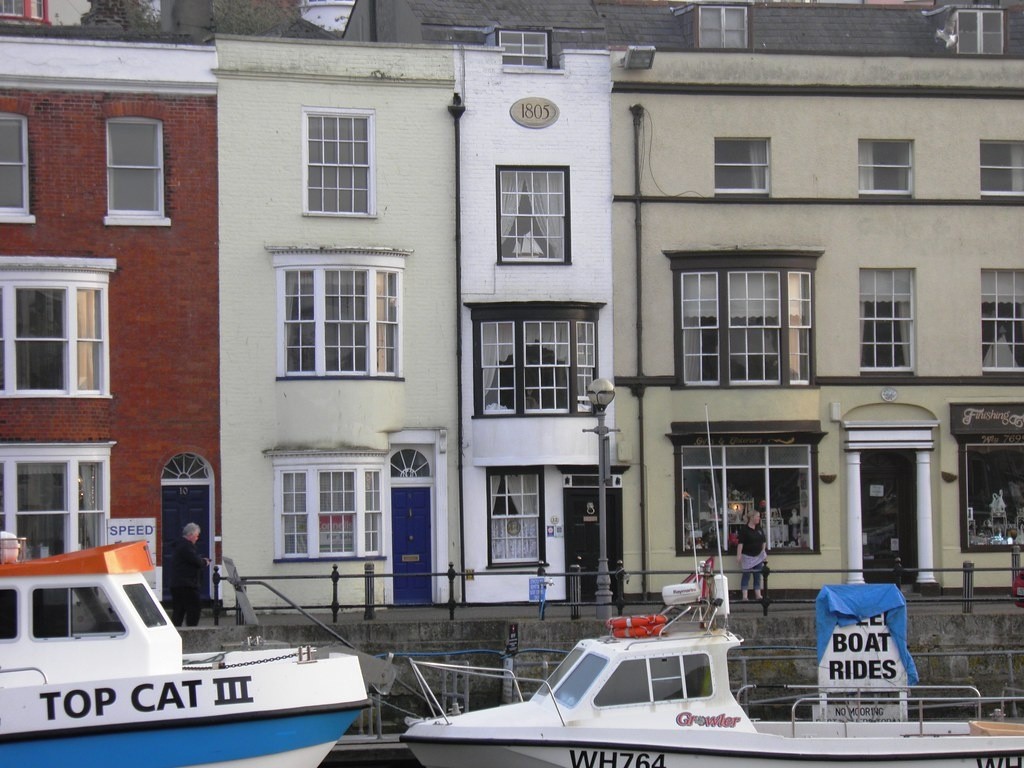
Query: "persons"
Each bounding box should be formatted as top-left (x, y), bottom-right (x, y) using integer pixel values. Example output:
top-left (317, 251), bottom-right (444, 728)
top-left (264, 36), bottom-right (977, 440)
top-left (737, 510), bottom-right (770, 601)
top-left (171, 522), bottom-right (212, 627)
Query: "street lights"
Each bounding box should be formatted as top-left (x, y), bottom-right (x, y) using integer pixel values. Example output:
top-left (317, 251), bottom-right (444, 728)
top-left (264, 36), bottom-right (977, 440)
top-left (581, 377), bottom-right (621, 623)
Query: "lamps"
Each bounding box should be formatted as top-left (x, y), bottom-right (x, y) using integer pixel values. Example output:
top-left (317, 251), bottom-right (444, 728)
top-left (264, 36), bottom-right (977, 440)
top-left (616, 45), bottom-right (656, 70)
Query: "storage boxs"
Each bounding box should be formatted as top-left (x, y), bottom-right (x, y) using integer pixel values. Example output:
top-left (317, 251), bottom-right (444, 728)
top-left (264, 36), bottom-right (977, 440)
top-left (968, 720), bottom-right (1024, 736)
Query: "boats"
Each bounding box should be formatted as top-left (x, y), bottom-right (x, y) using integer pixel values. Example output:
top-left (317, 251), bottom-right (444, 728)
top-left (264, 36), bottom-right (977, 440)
top-left (0, 531), bottom-right (397, 768)
top-left (399, 402), bottom-right (1024, 768)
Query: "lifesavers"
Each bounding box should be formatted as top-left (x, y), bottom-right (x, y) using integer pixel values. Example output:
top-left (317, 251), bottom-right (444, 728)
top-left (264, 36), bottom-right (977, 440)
top-left (605, 611), bottom-right (668, 638)
